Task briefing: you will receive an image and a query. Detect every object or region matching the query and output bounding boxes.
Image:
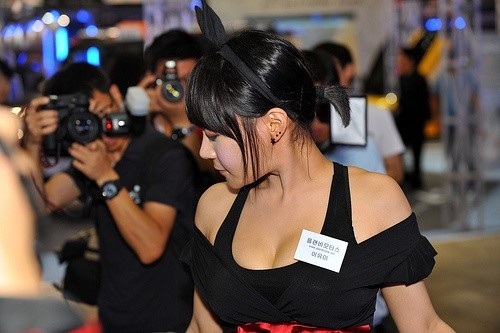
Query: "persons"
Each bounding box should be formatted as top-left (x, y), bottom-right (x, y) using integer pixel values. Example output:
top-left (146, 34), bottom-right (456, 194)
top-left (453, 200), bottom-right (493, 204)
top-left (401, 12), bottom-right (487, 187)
top-left (179, 0), bottom-right (456, 333)
top-left (298, 0), bottom-right (479, 333)
top-left (136, 28), bottom-right (227, 192)
top-left (25, 62), bottom-right (201, 333)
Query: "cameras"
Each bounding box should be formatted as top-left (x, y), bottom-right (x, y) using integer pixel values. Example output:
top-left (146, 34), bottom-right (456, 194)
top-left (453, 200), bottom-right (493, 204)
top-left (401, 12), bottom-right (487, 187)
top-left (42, 95), bottom-right (129, 157)
top-left (153, 58), bottom-right (183, 101)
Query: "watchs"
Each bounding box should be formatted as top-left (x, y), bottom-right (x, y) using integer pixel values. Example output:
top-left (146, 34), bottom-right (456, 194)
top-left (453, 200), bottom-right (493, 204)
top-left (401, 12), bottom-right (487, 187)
top-left (96, 178), bottom-right (126, 201)
top-left (172, 127), bottom-right (196, 141)
